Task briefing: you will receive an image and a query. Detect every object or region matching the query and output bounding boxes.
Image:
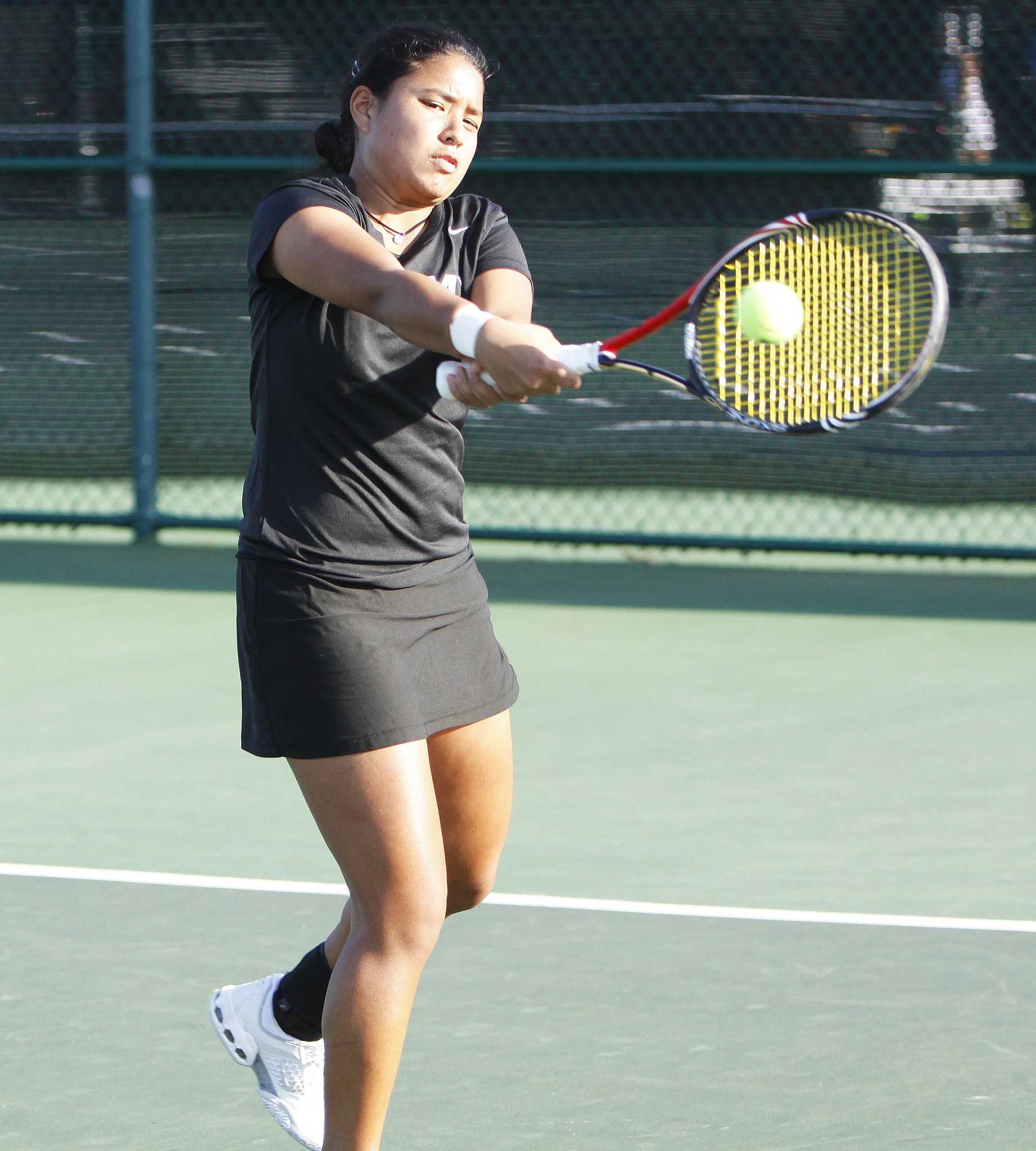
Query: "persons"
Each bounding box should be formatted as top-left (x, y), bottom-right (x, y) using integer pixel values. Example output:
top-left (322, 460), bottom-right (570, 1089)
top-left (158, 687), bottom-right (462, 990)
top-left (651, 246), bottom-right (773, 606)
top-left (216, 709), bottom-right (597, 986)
top-left (213, 24), bottom-right (582, 1150)
top-left (931, 13), bottom-right (1006, 236)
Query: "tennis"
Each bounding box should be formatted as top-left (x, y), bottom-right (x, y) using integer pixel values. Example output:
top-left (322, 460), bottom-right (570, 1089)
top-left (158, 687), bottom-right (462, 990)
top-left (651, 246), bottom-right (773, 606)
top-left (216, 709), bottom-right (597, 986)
top-left (733, 280), bottom-right (805, 345)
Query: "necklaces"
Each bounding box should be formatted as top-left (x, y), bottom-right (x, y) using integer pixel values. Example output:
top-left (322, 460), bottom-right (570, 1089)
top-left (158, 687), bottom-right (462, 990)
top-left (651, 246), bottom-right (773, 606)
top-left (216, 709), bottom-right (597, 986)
top-left (366, 208), bottom-right (428, 244)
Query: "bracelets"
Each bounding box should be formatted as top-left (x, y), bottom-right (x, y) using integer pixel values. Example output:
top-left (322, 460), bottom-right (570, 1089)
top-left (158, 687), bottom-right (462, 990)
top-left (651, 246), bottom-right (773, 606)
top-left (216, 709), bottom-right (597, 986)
top-left (450, 306), bottom-right (493, 359)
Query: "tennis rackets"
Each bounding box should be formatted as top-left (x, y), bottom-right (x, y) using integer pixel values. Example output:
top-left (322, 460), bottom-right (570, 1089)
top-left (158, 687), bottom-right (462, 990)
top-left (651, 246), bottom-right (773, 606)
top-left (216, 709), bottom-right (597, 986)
top-left (436, 208), bottom-right (950, 436)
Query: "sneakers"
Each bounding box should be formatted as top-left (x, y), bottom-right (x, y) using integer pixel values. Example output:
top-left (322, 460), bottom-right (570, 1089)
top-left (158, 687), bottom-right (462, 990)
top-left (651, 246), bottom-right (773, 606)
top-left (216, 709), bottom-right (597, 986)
top-left (208, 975), bottom-right (326, 1150)
